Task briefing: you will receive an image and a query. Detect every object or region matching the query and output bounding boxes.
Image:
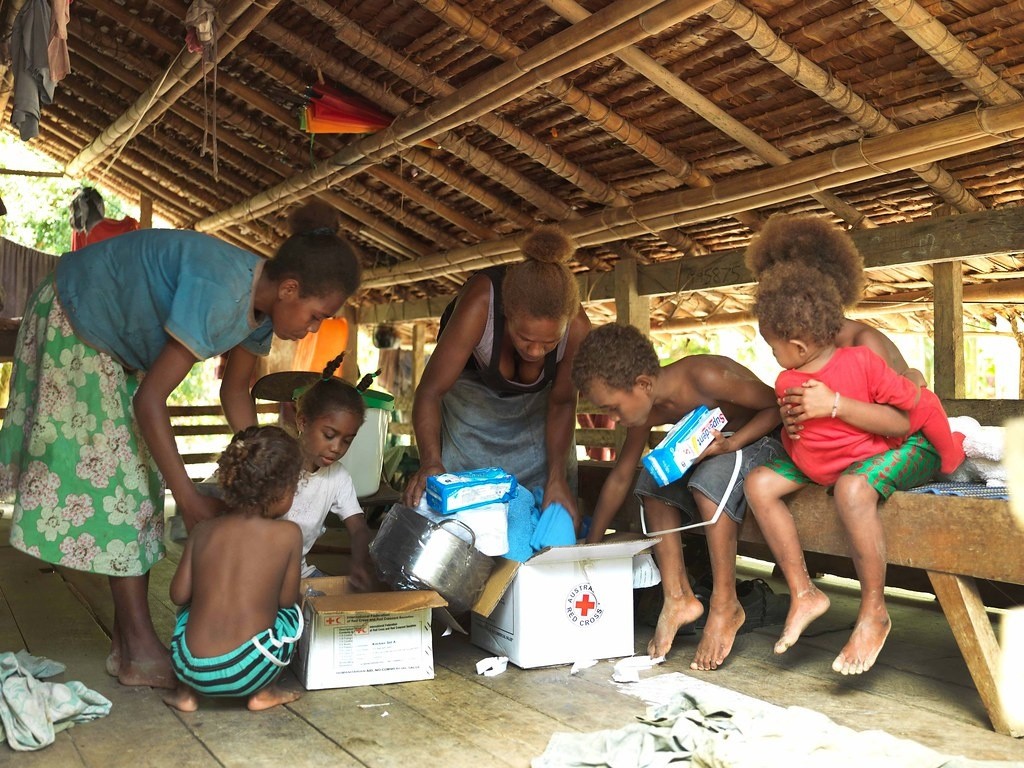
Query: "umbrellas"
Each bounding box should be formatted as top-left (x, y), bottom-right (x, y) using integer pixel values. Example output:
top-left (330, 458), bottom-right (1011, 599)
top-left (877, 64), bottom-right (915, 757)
top-left (297, 82), bottom-right (442, 150)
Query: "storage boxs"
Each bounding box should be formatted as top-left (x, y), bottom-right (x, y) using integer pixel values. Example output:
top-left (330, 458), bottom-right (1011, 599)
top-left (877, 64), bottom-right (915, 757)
top-left (470, 538), bottom-right (662, 668)
top-left (338, 390), bottom-right (396, 498)
top-left (287, 577), bottom-right (468, 691)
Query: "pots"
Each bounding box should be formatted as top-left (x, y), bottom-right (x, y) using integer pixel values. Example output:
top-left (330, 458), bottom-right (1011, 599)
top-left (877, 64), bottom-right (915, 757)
top-left (370, 503), bottom-right (494, 612)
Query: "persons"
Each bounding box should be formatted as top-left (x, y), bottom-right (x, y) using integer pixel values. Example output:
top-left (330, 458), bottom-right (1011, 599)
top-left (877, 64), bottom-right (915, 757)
top-left (742, 211), bottom-right (940, 676)
top-left (169, 349), bottom-right (384, 595)
top-left (0, 202), bottom-right (361, 689)
top-left (571, 322), bottom-right (788, 671)
top-left (160, 425), bottom-right (302, 712)
top-left (750, 262), bottom-right (966, 485)
top-left (402, 224), bottom-right (593, 535)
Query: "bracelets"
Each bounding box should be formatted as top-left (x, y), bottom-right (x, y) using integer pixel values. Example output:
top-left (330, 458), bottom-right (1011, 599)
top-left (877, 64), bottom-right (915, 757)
top-left (831, 392), bottom-right (840, 418)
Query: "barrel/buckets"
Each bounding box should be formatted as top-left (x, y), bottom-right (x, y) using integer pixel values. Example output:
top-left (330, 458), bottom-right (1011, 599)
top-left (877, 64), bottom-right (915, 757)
top-left (337, 408), bottom-right (394, 498)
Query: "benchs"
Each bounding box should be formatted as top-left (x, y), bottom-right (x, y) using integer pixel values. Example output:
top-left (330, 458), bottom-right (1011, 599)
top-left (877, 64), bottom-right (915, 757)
top-left (578, 400), bottom-right (1024, 740)
